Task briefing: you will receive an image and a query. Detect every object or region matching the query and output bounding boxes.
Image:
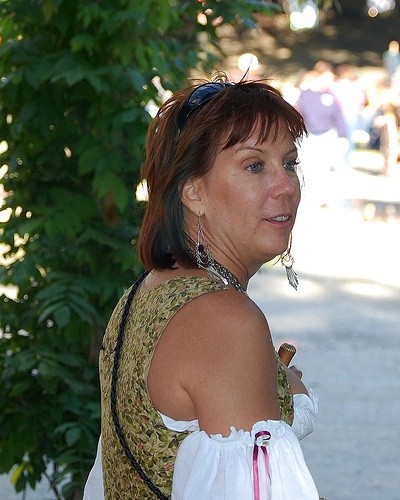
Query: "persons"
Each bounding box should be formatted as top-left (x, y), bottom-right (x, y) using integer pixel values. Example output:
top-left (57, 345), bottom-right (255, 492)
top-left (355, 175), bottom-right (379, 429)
top-left (195, 8), bottom-right (399, 193)
top-left (99, 67), bottom-right (321, 499)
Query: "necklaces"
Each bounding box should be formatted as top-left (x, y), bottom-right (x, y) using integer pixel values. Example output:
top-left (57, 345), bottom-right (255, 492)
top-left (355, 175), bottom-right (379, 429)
top-left (179, 230), bottom-right (250, 299)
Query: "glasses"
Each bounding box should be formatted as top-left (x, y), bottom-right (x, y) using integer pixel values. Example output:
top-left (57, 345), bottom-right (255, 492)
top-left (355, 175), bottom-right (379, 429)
top-left (175, 82), bottom-right (236, 137)
